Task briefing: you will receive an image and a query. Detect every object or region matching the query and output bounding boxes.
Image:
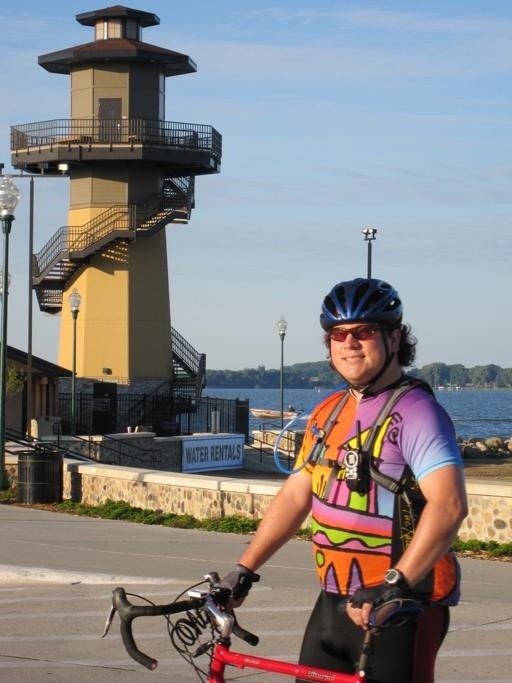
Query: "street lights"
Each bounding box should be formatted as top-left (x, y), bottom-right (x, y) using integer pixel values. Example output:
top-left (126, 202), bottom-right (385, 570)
top-left (68, 287), bottom-right (81, 434)
top-left (1, 174), bottom-right (22, 492)
top-left (277, 315), bottom-right (288, 429)
top-left (361, 227), bottom-right (376, 277)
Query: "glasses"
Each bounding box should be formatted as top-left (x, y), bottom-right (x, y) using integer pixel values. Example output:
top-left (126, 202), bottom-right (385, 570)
top-left (324, 324), bottom-right (383, 342)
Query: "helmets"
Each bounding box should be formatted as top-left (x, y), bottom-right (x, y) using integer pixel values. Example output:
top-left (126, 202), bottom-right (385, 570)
top-left (319, 278), bottom-right (404, 327)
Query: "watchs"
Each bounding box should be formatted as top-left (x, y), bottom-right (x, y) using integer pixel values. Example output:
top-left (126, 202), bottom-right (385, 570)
top-left (381, 568), bottom-right (412, 598)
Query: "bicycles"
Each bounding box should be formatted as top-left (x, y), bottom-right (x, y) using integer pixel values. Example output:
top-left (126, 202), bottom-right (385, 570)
top-left (100, 572), bottom-right (421, 683)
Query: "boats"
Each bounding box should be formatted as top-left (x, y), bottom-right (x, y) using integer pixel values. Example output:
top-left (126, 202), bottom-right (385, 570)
top-left (248, 408), bottom-right (303, 419)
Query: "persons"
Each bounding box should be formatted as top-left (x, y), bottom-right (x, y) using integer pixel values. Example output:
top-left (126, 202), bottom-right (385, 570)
top-left (209, 275), bottom-right (473, 681)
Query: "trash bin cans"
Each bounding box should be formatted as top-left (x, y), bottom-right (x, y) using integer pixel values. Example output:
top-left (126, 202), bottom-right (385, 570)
top-left (18, 450), bottom-right (64, 505)
